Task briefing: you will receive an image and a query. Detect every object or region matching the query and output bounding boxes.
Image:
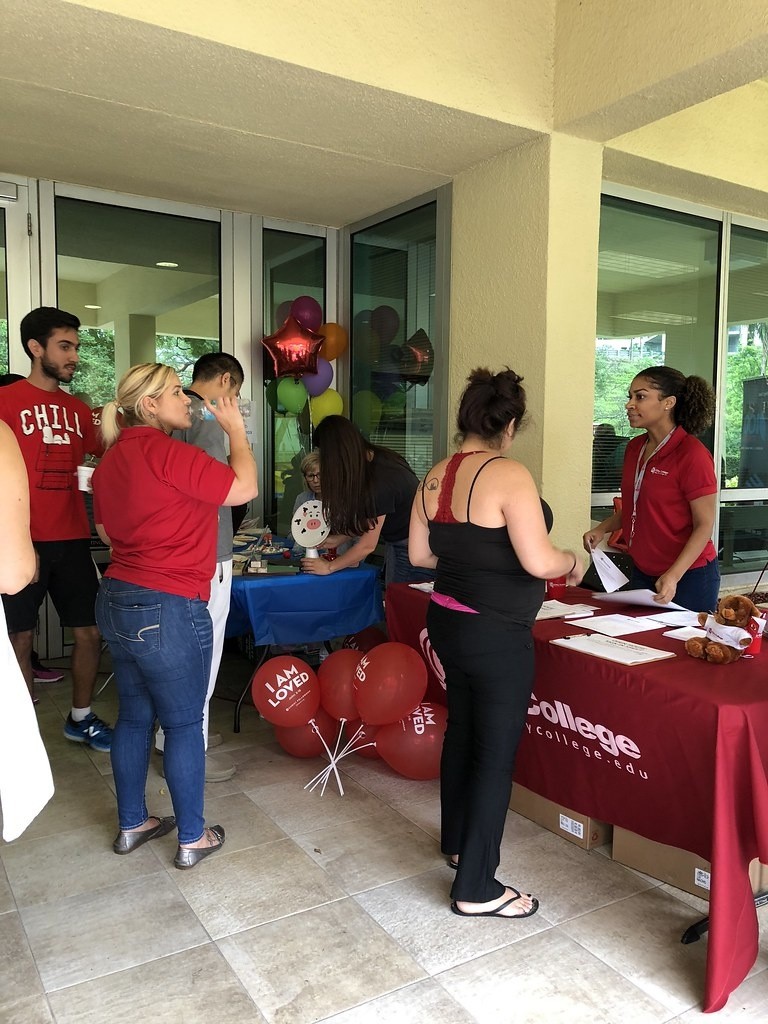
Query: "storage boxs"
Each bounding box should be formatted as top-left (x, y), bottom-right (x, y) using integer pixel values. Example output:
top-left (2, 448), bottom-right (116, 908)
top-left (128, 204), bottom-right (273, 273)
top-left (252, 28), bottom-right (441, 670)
top-left (504, 776), bottom-right (768, 902)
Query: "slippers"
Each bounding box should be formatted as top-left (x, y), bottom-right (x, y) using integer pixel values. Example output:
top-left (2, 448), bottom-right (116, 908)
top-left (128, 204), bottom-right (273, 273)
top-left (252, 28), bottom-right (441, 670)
top-left (448, 853), bottom-right (460, 870)
top-left (451, 886), bottom-right (539, 918)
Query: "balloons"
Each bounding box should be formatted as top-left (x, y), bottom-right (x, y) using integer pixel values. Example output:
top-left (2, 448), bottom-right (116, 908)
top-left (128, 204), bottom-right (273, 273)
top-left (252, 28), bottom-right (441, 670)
top-left (289, 296), bottom-right (322, 331)
top-left (277, 377), bottom-right (307, 415)
top-left (318, 324), bottom-right (348, 362)
top-left (301, 357), bottom-right (333, 397)
top-left (260, 314), bottom-right (326, 377)
top-left (320, 641), bottom-right (449, 781)
top-left (252, 655), bottom-right (339, 757)
top-left (311, 388), bottom-right (343, 430)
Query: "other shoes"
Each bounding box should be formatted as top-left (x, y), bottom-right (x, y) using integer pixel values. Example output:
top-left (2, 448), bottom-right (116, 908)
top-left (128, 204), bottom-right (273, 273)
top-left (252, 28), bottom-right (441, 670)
top-left (113, 816), bottom-right (177, 855)
top-left (174, 823), bottom-right (225, 869)
top-left (207, 728), bottom-right (224, 747)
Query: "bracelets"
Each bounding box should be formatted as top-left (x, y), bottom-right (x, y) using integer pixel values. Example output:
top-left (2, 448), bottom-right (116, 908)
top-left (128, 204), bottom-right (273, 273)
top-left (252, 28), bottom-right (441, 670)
top-left (567, 552), bottom-right (577, 573)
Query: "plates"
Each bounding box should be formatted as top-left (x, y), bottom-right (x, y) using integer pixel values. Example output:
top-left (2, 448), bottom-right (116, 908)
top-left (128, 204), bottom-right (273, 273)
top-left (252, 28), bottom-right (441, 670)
top-left (235, 535), bottom-right (257, 541)
top-left (234, 541), bottom-right (247, 546)
top-left (244, 528), bottom-right (271, 534)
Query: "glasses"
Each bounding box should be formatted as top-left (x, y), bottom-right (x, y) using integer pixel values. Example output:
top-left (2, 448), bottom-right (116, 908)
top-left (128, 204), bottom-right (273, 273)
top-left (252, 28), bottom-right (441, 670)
top-left (220, 373), bottom-right (242, 403)
top-left (305, 472), bottom-right (322, 483)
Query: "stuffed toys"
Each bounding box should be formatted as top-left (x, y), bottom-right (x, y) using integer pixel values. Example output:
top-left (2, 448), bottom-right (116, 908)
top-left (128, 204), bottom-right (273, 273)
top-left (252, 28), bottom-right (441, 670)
top-left (685, 595), bottom-right (761, 665)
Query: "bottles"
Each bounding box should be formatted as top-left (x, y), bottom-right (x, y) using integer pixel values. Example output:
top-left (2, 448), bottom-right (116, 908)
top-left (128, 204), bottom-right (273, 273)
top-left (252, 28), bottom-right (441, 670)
top-left (322, 548), bottom-right (340, 562)
top-left (187, 398), bottom-right (250, 421)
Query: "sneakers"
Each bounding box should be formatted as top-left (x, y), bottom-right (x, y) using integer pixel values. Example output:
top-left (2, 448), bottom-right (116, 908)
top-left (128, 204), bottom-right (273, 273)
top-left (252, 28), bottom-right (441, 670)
top-left (31, 662), bottom-right (64, 682)
top-left (63, 709), bottom-right (115, 753)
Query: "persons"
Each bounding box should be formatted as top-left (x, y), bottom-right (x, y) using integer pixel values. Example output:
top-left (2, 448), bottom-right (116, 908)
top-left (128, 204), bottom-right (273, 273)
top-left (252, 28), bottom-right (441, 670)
top-left (155, 351), bottom-right (245, 783)
top-left (582, 367), bottom-right (720, 614)
top-left (91, 363), bottom-right (260, 869)
top-left (0, 374), bottom-right (65, 683)
top-left (300, 416), bottom-right (435, 585)
top-left (0, 420), bottom-right (56, 843)
top-left (349, 309), bottom-right (436, 432)
top-left (0, 305), bottom-right (120, 752)
top-left (291, 450), bottom-right (368, 564)
top-left (408, 366), bottom-right (583, 918)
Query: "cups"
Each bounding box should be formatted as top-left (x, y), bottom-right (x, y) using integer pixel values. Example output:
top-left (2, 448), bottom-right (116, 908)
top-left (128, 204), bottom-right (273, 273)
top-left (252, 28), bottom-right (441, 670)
top-left (547, 576), bottom-right (566, 600)
top-left (77, 465), bottom-right (95, 492)
top-left (744, 630), bottom-right (762, 654)
top-left (306, 547), bottom-right (320, 559)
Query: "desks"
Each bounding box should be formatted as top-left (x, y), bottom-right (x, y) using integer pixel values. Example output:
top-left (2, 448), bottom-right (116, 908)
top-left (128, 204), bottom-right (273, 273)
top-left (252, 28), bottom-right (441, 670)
top-left (387, 579), bottom-right (768, 1015)
top-left (231, 564), bottom-right (383, 733)
